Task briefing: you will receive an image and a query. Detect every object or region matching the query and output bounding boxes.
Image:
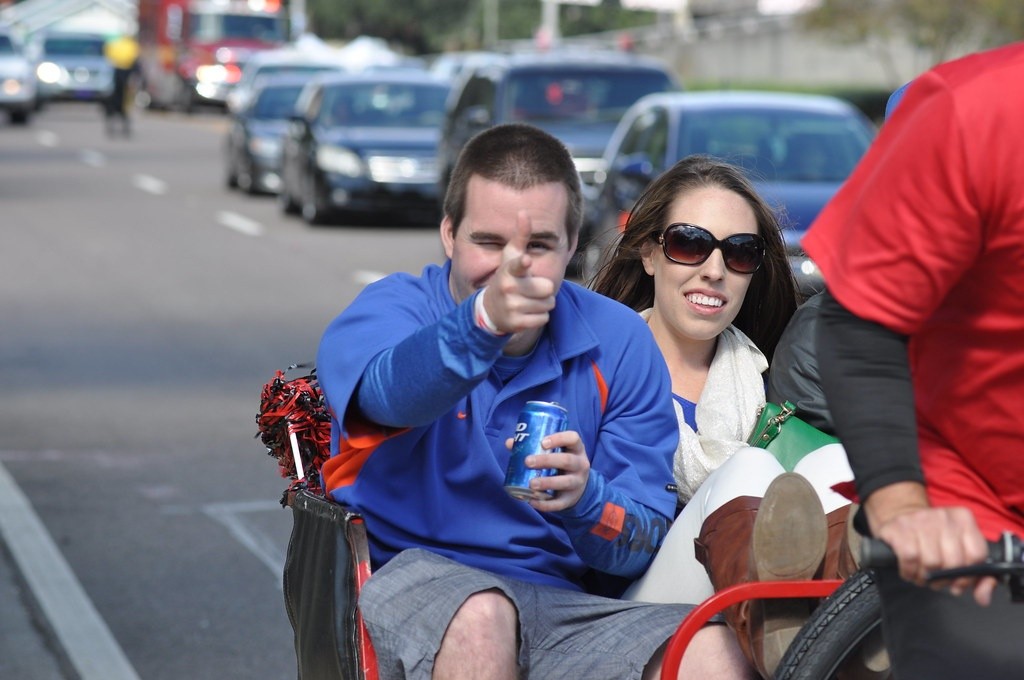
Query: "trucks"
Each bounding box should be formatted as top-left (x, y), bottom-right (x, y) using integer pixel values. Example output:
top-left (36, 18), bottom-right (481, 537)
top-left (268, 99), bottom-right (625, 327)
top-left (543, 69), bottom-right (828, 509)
top-left (136, 0), bottom-right (291, 115)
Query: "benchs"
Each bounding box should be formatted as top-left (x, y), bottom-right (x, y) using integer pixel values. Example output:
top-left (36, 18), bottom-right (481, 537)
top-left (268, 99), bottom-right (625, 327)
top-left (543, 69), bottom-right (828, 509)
top-left (279, 361), bottom-right (377, 680)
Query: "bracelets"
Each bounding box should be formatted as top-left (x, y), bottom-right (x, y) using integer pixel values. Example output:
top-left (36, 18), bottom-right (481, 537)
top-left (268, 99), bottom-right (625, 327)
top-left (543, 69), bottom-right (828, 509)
top-left (475, 286), bottom-right (506, 336)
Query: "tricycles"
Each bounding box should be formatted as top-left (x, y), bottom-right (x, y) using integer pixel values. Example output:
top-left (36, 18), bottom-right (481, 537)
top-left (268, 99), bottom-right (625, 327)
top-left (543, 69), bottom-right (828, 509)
top-left (275, 464), bottom-right (1024, 680)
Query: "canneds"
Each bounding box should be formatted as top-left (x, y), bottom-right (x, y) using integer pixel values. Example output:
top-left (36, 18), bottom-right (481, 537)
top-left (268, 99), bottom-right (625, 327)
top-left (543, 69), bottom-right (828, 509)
top-left (503, 401), bottom-right (568, 501)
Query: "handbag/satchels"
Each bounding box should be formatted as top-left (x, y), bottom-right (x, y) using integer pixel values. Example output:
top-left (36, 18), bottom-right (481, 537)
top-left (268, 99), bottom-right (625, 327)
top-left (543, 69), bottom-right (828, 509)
top-left (747, 400), bottom-right (843, 472)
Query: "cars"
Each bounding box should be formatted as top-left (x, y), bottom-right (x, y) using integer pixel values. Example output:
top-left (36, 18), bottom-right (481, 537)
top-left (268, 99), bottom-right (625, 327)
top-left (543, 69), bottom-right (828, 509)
top-left (286, 76), bottom-right (457, 226)
top-left (227, 78), bottom-right (318, 194)
top-left (0, 35), bottom-right (40, 122)
top-left (230, 57), bottom-right (348, 114)
top-left (587, 90), bottom-right (883, 318)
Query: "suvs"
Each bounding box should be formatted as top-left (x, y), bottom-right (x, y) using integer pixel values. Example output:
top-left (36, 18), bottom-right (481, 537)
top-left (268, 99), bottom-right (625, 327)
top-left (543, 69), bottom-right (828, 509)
top-left (37, 32), bottom-right (123, 104)
top-left (444, 53), bottom-right (680, 251)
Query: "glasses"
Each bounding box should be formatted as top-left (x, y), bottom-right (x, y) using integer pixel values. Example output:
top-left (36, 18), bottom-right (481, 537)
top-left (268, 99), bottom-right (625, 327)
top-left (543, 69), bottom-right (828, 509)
top-left (649, 222), bottom-right (765, 274)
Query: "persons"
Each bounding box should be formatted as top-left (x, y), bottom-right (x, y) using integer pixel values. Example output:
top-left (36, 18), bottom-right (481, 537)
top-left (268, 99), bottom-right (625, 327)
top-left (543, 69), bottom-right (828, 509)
top-left (318, 123), bottom-right (761, 680)
top-left (767, 40), bottom-right (1024, 680)
top-left (592, 154), bottom-right (856, 604)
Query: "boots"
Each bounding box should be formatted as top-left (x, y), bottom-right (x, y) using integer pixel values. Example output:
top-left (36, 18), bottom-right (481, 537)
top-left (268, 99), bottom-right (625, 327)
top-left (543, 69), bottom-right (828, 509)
top-left (694, 472), bottom-right (828, 680)
top-left (811, 502), bottom-right (891, 672)
